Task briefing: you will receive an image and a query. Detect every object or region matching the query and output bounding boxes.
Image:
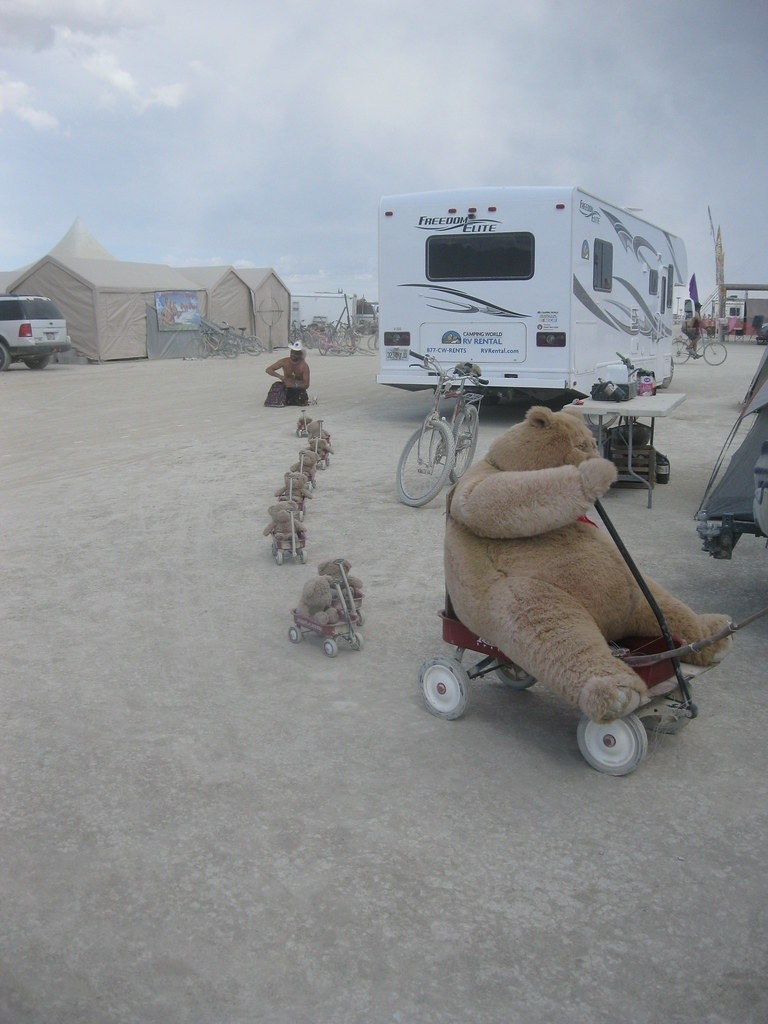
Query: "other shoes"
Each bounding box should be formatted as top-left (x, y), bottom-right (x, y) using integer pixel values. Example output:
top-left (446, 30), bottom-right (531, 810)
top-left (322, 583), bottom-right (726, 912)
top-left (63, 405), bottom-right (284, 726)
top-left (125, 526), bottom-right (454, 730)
top-left (690, 352), bottom-right (696, 356)
top-left (694, 354), bottom-right (703, 358)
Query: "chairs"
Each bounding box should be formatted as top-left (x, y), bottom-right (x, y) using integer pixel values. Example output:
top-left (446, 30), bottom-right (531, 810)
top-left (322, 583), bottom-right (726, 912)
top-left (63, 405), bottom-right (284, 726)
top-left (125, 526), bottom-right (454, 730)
top-left (734, 329), bottom-right (744, 343)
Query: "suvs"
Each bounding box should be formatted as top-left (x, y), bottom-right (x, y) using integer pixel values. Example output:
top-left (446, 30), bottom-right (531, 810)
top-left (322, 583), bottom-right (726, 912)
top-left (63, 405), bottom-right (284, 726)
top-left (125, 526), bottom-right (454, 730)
top-left (0, 294), bottom-right (72, 372)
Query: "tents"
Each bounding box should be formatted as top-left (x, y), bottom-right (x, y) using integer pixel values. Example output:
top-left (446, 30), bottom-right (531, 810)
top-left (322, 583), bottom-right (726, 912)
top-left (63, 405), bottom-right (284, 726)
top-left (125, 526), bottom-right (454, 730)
top-left (693, 343), bottom-right (768, 520)
top-left (9, 254), bottom-right (291, 364)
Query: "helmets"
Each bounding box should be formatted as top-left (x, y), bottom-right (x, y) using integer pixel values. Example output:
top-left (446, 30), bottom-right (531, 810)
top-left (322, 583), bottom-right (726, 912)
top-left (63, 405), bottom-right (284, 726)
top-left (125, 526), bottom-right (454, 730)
top-left (454, 363), bottom-right (481, 377)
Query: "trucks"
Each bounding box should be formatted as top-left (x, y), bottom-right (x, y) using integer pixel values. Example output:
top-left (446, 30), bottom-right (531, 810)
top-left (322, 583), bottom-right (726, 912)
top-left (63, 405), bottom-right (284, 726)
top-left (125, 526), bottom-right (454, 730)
top-left (377, 184), bottom-right (697, 407)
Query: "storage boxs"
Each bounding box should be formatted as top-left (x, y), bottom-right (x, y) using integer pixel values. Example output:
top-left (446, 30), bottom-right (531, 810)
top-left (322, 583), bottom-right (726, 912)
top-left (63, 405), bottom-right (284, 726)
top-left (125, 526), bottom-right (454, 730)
top-left (609, 445), bottom-right (657, 490)
top-left (589, 381), bottom-right (638, 401)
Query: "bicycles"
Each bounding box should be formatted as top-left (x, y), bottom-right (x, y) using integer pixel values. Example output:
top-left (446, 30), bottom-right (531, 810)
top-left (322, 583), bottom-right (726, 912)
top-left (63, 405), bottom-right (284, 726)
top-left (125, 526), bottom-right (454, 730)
top-left (395, 349), bottom-right (489, 507)
top-left (289, 314), bottom-right (377, 357)
top-left (670, 329), bottom-right (728, 366)
top-left (192, 317), bottom-right (266, 359)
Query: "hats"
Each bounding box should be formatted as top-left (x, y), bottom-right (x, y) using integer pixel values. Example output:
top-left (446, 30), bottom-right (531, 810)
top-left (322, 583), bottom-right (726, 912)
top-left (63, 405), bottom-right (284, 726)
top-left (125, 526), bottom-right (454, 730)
top-left (289, 342), bottom-right (304, 351)
top-left (695, 302), bottom-right (703, 310)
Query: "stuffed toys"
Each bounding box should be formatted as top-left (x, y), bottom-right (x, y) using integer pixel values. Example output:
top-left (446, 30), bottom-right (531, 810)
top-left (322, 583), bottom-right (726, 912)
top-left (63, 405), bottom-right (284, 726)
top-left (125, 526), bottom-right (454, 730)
top-left (296, 559), bottom-right (363, 625)
top-left (262, 418), bottom-right (335, 540)
top-left (445, 406), bottom-right (736, 725)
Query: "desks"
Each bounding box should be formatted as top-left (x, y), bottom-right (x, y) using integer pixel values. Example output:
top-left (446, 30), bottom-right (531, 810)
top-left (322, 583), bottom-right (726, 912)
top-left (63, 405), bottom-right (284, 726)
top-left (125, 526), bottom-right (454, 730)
top-left (564, 392), bottom-right (686, 508)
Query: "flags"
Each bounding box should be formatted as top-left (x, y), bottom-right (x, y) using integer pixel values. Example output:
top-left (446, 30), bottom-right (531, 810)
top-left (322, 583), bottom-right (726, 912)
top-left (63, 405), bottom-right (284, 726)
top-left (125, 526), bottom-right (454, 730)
top-left (689, 274), bottom-right (698, 302)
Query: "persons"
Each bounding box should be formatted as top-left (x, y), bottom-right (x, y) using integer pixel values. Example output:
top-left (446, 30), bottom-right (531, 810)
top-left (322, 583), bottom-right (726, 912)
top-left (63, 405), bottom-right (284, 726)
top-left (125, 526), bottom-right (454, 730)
top-left (266, 340), bottom-right (310, 406)
top-left (681, 302), bottom-right (703, 360)
